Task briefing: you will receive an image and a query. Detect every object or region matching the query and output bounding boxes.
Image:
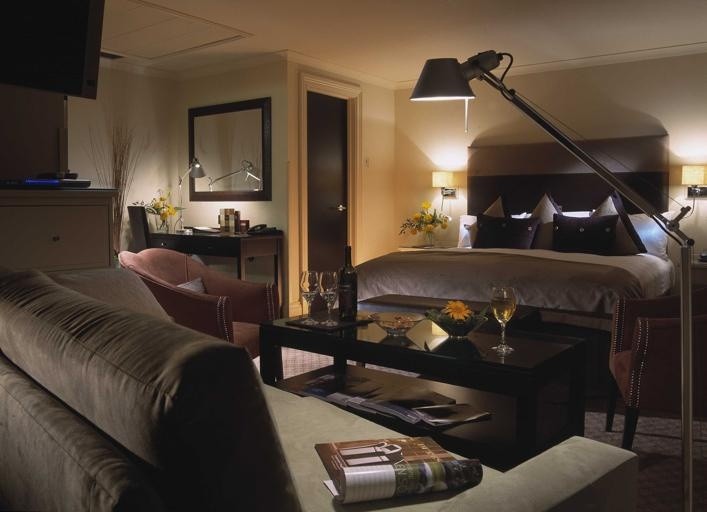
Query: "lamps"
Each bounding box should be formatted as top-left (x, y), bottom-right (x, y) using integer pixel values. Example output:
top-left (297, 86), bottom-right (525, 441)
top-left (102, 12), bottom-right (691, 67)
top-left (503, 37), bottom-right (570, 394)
top-left (178, 158), bottom-right (206, 235)
top-left (208, 159), bottom-right (262, 191)
top-left (409, 48), bottom-right (693, 512)
top-left (432, 170), bottom-right (458, 201)
top-left (682, 166), bottom-right (707, 198)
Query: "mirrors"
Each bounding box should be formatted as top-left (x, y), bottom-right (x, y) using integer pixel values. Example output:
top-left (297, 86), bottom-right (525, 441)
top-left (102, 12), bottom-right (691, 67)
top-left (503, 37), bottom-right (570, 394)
top-left (188, 96), bottom-right (273, 201)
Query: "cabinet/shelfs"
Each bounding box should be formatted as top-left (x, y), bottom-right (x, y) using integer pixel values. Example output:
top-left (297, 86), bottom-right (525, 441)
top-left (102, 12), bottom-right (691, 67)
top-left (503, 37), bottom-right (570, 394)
top-left (679, 253), bottom-right (707, 289)
top-left (0, 190), bottom-right (118, 270)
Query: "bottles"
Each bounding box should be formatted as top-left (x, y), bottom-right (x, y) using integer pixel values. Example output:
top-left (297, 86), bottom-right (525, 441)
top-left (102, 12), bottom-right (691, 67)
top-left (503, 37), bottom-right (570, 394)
top-left (338, 245), bottom-right (358, 322)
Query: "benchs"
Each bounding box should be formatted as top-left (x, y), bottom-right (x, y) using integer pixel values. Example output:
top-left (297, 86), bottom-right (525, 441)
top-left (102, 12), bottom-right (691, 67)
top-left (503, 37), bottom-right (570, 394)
top-left (359, 295), bottom-right (542, 342)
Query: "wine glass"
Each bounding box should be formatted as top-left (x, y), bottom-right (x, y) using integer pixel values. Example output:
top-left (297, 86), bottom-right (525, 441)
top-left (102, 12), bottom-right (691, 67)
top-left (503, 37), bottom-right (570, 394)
top-left (489, 286), bottom-right (516, 354)
top-left (319, 270), bottom-right (340, 328)
top-left (299, 270), bottom-right (319, 326)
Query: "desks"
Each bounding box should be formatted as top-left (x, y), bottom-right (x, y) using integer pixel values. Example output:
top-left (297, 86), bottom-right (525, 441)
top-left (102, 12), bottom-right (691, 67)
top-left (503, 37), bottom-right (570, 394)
top-left (147, 230), bottom-right (284, 319)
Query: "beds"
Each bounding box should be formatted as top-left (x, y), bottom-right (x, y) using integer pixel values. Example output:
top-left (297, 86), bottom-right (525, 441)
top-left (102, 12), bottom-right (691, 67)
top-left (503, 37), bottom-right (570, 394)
top-left (335, 135), bottom-right (677, 353)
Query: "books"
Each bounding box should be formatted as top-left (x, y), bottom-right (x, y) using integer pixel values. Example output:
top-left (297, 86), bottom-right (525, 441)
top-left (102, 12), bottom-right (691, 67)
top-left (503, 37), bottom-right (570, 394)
top-left (311, 434), bottom-right (485, 505)
top-left (303, 368), bottom-right (493, 431)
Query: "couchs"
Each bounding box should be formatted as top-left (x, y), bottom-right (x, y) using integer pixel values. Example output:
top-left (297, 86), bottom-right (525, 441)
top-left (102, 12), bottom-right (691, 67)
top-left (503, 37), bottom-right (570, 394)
top-left (0, 268), bottom-right (645, 512)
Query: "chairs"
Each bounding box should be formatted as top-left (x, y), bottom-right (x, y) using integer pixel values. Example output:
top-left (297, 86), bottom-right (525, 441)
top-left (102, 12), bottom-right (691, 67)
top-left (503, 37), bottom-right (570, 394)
top-left (127, 206), bottom-right (151, 253)
top-left (116, 246), bottom-right (280, 360)
top-left (607, 280), bottom-right (707, 450)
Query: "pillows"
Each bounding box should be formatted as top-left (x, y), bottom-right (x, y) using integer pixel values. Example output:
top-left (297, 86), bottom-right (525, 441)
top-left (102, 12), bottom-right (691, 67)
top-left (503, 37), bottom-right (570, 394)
top-left (177, 275), bottom-right (207, 294)
top-left (458, 191), bottom-right (673, 259)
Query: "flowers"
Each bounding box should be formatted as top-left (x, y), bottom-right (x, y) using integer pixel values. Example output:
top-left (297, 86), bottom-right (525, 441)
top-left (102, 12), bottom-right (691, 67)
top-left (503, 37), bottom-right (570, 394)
top-left (133, 189), bottom-right (176, 226)
top-left (398, 201), bottom-right (449, 239)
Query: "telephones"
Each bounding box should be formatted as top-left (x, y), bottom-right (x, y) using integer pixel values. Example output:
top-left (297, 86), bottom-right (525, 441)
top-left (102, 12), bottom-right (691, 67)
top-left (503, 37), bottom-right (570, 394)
top-left (247, 224), bottom-right (277, 235)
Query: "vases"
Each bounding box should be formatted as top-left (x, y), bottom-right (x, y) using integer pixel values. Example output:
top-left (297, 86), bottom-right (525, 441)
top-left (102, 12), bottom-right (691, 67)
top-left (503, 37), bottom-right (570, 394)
top-left (154, 214), bottom-right (174, 230)
top-left (427, 233), bottom-right (434, 244)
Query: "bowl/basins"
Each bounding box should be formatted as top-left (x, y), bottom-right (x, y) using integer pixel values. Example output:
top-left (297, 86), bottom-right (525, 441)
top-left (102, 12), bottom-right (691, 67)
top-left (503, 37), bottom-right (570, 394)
top-left (427, 314), bottom-right (489, 339)
top-left (367, 311), bottom-right (426, 336)
top-left (373, 336), bottom-right (423, 350)
top-left (429, 339), bottom-right (483, 357)
